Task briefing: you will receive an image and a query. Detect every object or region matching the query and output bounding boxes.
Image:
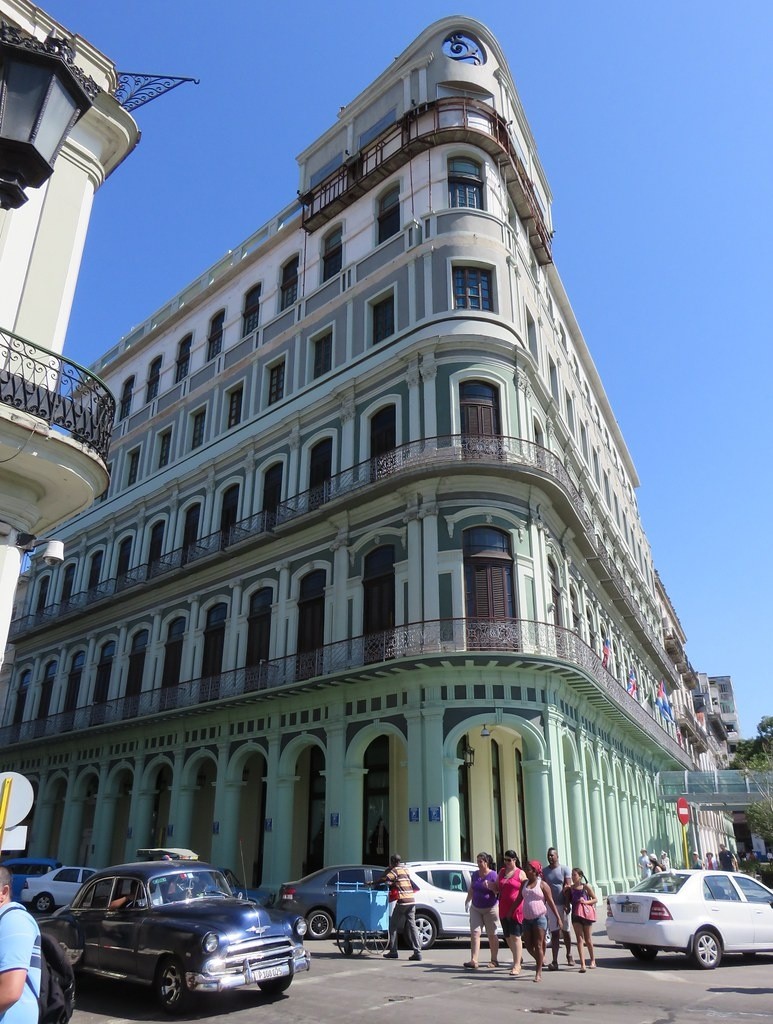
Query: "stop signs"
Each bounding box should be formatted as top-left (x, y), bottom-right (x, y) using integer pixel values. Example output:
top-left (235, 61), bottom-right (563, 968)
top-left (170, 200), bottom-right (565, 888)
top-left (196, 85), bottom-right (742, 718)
top-left (677, 797), bottom-right (690, 825)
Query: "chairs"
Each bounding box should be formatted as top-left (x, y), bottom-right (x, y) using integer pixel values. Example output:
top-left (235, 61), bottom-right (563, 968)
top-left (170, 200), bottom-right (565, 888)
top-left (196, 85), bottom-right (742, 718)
top-left (708, 886), bottom-right (729, 900)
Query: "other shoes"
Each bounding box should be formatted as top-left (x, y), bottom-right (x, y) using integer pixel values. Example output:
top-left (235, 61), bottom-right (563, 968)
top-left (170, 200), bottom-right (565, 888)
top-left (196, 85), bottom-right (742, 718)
top-left (589, 961), bottom-right (595, 968)
top-left (463, 961), bottom-right (478, 968)
top-left (409, 954), bottom-right (422, 961)
top-left (383, 953), bottom-right (398, 959)
top-left (567, 956), bottom-right (575, 966)
top-left (488, 960), bottom-right (499, 969)
top-left (548, 962), bottom-right (560, 971)
top-left (510, 963), bottom-right (523, 975)
top-left (520, 958), bottom-right (524, 964)
top-left (579, 965), bottom-right (585, 972)
top-left (534, 975), bottom-right (540, 982)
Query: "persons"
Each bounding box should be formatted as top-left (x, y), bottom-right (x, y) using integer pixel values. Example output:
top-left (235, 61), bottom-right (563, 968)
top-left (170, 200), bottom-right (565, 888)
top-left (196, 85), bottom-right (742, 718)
top-left (649, 850), bottom-right (670, 875)
top-left (366, 854), bottom-right (422, 961)
top-left (110, 879), bottom-right (145, 909)
top-left (767, 850), bottom-right (773, 865)
top-left (738, 850), bottom-right (758, 862)
top-left (463, 852), bottom-right (498, 968)
top-left (637, 849), bottom-right (653, 881)
top-left (483, 850), bottom-right (529, 975)
top-left (507, 861), bottom-right (563, 982)
top-left (0, 865), bottom-right (42, 1024)
top-left (692, 844), bottom-right (738, 872)
top-left (563, 868), bottom-right (598, 972)
top-left (542, 847), bottom-right (575, 971)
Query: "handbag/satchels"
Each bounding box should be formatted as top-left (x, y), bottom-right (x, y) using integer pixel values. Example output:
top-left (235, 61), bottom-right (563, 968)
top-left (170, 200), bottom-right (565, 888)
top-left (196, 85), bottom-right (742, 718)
top-left (574, 886), bottom-right (596, 922)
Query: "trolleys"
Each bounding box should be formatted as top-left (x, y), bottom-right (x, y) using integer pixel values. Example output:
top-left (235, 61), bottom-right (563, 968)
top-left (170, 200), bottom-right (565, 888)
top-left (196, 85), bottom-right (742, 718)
top-left (335, 872), bottom-right (398, 958)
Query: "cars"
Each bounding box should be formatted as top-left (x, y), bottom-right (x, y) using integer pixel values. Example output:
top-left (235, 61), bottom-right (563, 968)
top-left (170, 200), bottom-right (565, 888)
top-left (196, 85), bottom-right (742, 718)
top-left (605, 869), bottom-right (773, 969)
top-left (402, 861), bottom-right (553, 950)
top-left (150, 868), bottom-right (276, 907)
top-left (276, 864), bottom-right (387, 940)
top-left (21, 865), bottom-right (100, 913)
top-left (0, 857), bottom-right (62, 903)
top-left (50, 848), bottom-right (312, 1011)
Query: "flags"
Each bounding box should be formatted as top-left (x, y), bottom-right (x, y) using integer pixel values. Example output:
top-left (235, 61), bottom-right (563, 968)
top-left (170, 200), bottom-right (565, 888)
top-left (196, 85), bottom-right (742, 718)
top-left (654, 677), bottom-right (675, 725)
top-left (677, 722), bottom-right (682, 747)
top-left (626, 666), bottom-right (638, 698)
top-left (602, 638), bottom-right (610, 669)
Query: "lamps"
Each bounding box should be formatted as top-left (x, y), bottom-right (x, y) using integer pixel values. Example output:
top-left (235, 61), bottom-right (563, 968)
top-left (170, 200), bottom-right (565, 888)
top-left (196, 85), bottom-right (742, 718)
top-left (297, 190), bottom-right (300, 195)
top-left (481, 724), bottom-right (490, 736)
top-left (411, 99), bottom-right (416, 106)
top-left (507, 120), bottom-right (513, 126)
top-left (462, 731), bottom-right (475, 768)
top-left (345, 149), bottom-right (350, 156)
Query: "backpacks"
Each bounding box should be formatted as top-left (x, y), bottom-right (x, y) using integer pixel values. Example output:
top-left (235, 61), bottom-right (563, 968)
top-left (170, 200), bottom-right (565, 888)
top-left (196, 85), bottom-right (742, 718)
top-left (1, 908), bottom-right (76, 1024)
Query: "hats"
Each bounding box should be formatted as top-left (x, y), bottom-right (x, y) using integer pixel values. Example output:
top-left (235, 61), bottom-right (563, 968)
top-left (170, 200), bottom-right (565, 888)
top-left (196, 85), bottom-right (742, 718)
top-left (640, 849), bottom-right (647, 853)
top-left (706, 853), bottom-right (713, 857)
top-left (649, 854), bottom-right (657, 861)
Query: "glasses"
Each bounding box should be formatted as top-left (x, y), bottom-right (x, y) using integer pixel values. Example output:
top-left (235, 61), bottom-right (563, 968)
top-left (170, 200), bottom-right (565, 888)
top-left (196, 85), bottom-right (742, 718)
top-left (504, 858), bottom-right (515, 862)
